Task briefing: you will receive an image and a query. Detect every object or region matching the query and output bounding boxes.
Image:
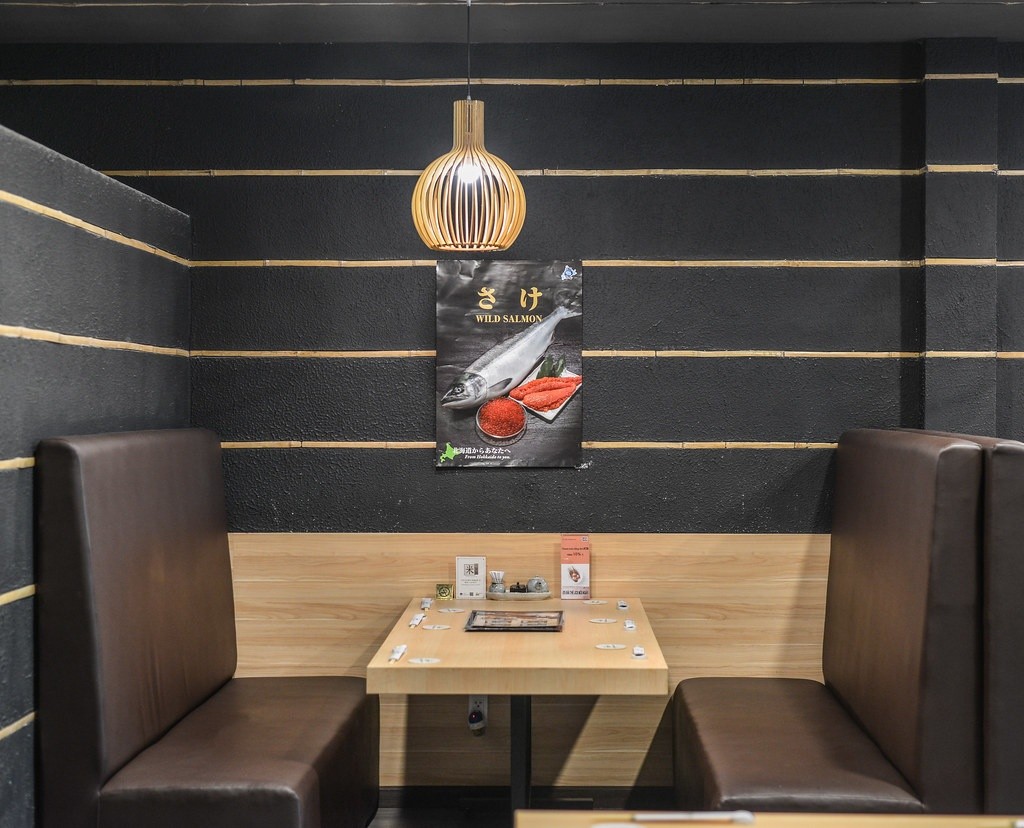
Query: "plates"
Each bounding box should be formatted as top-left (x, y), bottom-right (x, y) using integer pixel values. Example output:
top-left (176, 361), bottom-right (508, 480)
top-left (486, 590), bottom-right (552, 601)
top-left (508, 359), bottom-right (582, 420)
top-left (476, 398), bottom-right (528, 438)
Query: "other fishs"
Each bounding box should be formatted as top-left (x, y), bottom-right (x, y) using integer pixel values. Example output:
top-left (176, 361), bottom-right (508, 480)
top-left (441, 299), bottom-right (582, 410)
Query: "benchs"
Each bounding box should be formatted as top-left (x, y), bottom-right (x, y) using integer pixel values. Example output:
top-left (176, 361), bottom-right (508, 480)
top-left (672, 429), bottom-right (1024, 817)
top-left (33, 425), bottom-right (375, 828)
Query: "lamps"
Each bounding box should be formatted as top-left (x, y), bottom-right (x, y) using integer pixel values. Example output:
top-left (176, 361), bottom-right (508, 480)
top-left (410, 0), bottom-right (527, 253)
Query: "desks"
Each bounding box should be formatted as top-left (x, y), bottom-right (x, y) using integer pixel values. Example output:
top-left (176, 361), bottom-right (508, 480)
top-left (365, 596), bottom-right (670, 818)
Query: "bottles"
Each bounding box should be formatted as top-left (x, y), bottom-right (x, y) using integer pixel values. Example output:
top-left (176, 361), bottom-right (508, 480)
top-left (489, 582), bottom-right (505, 593)
top-left (510, 582), bottom-right (526, 593)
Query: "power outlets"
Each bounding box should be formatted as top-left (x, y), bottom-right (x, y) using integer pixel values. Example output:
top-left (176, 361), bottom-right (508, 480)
top-left (466, 695), bottom-right (488, 723)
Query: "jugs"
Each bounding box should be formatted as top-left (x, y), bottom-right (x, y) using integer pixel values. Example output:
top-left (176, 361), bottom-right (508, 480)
top-left (527, 575), bottom-right (549, 593)
